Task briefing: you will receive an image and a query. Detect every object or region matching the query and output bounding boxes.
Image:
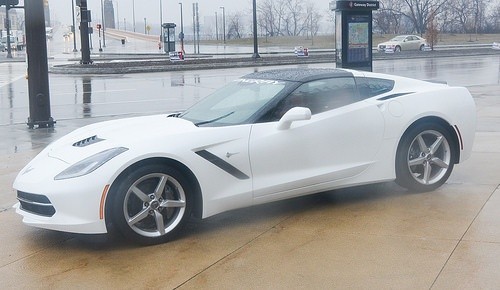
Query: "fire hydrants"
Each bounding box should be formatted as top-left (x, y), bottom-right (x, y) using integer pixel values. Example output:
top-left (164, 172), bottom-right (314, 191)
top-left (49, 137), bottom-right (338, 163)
top-left (179, 51), bottom-right (183, 59)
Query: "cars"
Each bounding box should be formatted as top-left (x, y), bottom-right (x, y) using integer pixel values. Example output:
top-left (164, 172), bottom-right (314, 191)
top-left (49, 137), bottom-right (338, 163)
top-left (378, 35), bottom-right (426, 53)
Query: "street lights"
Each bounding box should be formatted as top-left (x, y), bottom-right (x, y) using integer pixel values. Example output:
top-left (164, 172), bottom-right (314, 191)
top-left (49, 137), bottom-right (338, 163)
top-left (219, 7), bottom-right (225, 41)
top-left (178, 3), bottom-right (183, 51)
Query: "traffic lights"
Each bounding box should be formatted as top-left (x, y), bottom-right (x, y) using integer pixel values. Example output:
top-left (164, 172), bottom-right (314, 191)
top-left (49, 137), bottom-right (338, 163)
top-left (97, 24), bottom-right (102, 29)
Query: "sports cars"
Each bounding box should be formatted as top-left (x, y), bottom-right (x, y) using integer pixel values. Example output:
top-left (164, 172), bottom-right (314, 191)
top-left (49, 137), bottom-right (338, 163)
top-left (14, 69), bottom-right (477, 245)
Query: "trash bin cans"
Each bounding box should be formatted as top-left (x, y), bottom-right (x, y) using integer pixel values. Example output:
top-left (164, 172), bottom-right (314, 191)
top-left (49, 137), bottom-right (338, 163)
top-left (121, 39), bottom-right (125, 44)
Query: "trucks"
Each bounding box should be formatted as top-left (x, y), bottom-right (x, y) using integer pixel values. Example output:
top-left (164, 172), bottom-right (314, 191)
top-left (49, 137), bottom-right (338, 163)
top-left (45, 26), bottom-right (54, 39)
top-left (1, 30), bottom-right (26, 51)
